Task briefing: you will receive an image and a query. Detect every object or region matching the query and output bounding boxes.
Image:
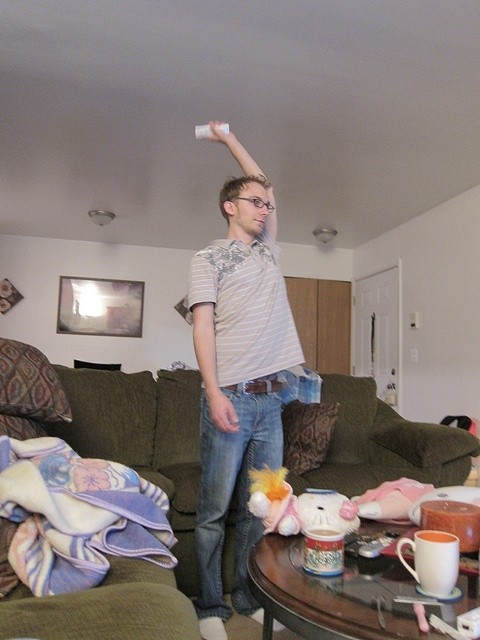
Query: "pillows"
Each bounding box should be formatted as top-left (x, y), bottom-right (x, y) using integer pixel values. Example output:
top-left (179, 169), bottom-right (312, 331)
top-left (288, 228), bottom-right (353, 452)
top-left (312, 373), bottom-right (376, 464)
top-left (45, 364), bottom-right (158, 466)
top-left (0, 337), bottom-right (74, 423)
top-left (0, 520), bottom-right (21, 601)
top-left (372, 416), bottom-right (480, 468)
top-left (283, 399), bottom-right (340, 475)
top-left (154, 368), bottom-right (203, 470)
top-left (0, 414), bottom-right (52, 442)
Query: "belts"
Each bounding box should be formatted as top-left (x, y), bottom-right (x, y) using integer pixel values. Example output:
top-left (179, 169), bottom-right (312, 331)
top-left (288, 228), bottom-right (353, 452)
top-left (223, 381), bottom-right (283, 393)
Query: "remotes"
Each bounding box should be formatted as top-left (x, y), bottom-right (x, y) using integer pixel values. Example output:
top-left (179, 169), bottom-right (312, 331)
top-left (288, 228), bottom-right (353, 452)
top-left (356, 535), bottom-right (398, 559)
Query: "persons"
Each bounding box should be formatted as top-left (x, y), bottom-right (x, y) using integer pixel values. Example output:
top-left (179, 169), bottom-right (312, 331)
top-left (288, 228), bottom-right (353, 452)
top-left (186, 120), bottom-right (307, 640)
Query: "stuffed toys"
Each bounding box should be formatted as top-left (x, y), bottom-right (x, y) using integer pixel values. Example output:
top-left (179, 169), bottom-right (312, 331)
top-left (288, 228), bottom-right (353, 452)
top-left (350, 477), bottom-right (480, 527)
top-left (247, 461), bottom-right (361, 537)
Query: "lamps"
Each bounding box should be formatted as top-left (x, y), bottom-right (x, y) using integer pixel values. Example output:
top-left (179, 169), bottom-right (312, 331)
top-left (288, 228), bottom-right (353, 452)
top-left (312, 228), bottom-right (338, 244)
top-left (88, 209), bottom-right (116, 228)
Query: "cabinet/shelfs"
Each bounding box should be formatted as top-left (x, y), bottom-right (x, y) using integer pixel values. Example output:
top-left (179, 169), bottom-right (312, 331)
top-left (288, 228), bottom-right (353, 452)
top-left (283, 275), bottom-right (352, 374)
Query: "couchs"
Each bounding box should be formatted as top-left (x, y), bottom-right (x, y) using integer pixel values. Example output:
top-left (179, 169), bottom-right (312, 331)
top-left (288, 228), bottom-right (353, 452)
top-left (0, 391), bottom-right (480, 640)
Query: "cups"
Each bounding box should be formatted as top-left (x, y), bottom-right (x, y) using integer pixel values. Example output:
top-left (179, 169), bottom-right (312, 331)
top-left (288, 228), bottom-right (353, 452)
top-left (302, 525), bottom-right (345, 576)
top-left (396, 530), bottom-right (460, 595)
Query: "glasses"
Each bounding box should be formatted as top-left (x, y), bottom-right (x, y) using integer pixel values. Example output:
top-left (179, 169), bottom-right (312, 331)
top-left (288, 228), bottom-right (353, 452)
top-left (237, 198), bottom-right (275, 213)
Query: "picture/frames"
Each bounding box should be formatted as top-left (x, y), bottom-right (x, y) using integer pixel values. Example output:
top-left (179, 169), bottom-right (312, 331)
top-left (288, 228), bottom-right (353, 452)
top-left (56, 274), bottom-right (145, 339)
